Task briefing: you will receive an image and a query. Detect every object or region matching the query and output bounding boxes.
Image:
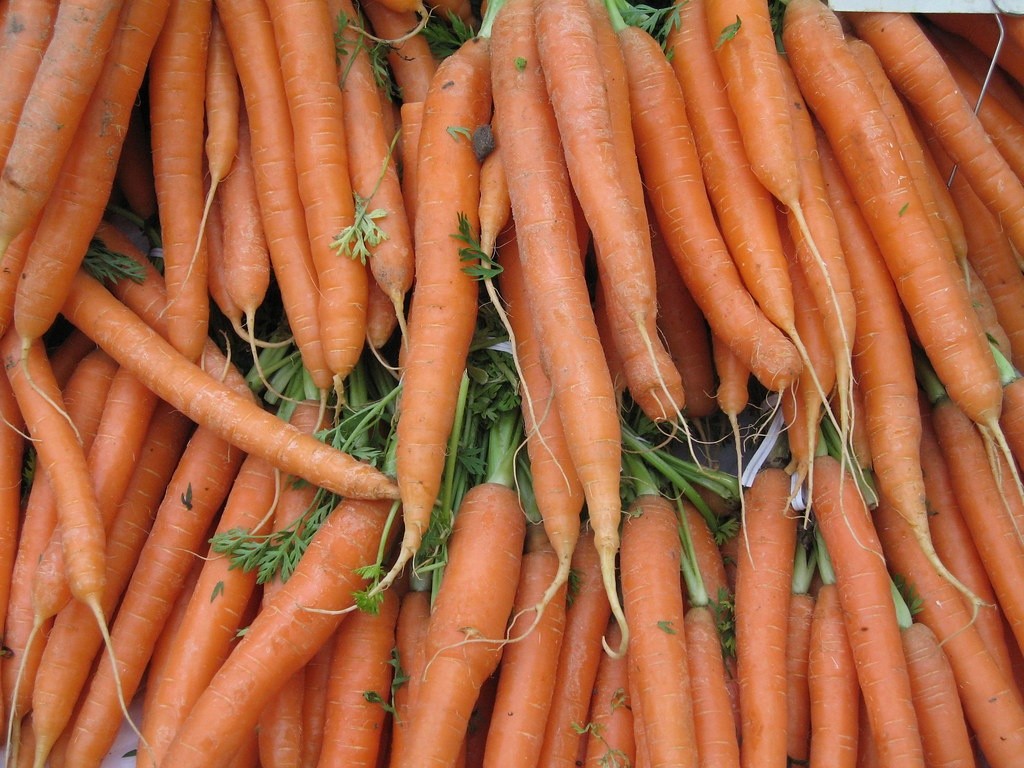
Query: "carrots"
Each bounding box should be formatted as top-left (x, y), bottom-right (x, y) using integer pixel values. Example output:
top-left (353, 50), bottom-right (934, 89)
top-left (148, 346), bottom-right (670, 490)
top-left (1, 1), bottom-right (1023, 768)
